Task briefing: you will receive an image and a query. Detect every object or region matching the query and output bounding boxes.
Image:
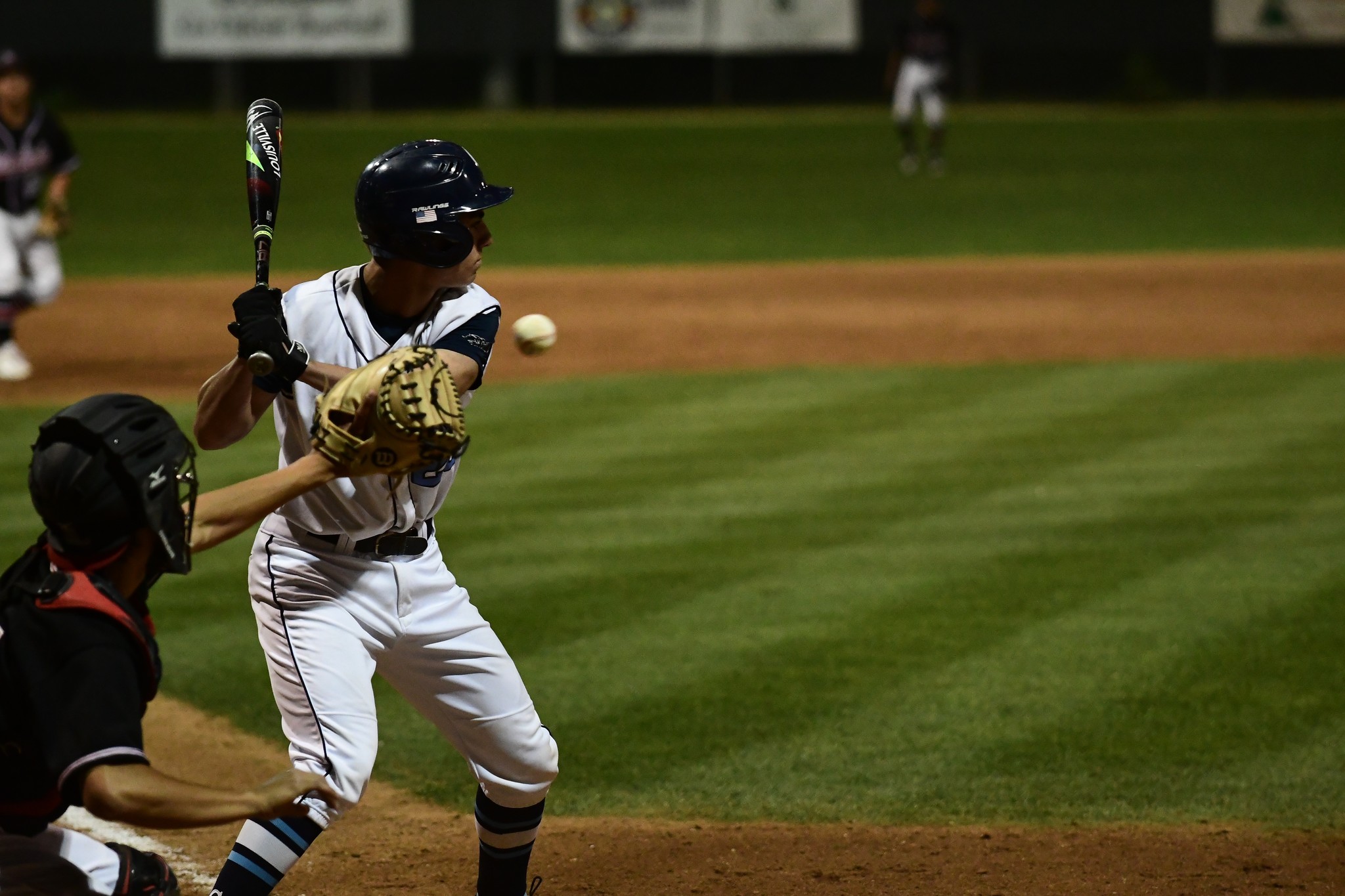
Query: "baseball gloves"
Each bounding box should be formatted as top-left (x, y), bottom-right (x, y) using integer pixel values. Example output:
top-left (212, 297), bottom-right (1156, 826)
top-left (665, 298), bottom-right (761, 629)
top-left (308, 343), bottom-right (467, 478)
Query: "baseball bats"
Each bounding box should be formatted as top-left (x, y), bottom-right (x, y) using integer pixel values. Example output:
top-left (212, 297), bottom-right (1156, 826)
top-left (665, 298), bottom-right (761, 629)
top-left (244, 96), bottom-right (283, 377)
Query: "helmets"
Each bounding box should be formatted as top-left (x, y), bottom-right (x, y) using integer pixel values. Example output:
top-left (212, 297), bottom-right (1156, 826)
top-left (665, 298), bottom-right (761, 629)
top-left (27, 422), bottom-right (145, 551)
top-left (355, 138), bottom-right (513, 275)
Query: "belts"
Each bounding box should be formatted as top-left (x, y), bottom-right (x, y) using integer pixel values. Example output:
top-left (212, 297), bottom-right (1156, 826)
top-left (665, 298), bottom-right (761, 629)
top-left (307, 517), bottom-right (435, 556)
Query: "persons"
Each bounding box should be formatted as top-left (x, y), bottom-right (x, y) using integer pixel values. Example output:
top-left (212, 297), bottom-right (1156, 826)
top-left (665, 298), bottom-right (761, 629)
top-left (0, 51), bottom-right (74, 380)
top-left (203, 129), bottom-right (561, 896)
top-left (2, 345), bottom-right (469, 896)
top-left (882, 0), bottom-right (948, 180)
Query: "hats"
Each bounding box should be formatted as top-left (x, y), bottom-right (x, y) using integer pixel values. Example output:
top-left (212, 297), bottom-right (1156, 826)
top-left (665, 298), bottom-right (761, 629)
top-left (0, 48), bottom-right (25, 80)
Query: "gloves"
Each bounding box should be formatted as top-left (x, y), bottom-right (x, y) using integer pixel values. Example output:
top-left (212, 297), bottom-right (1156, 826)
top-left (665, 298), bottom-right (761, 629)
top-left (226, 316), bottom-right (310, 395)
top-left (233, 287), bottom-right (288, 358)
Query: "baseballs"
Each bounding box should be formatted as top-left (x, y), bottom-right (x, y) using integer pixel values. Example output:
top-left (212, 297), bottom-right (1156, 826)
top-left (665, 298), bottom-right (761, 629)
top-left (509, 313), bottom-right (558, 356)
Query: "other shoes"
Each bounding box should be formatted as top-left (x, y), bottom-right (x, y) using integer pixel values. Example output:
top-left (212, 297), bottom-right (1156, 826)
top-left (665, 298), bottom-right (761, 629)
top-left (0, 340), bottom-right (30, 380)
